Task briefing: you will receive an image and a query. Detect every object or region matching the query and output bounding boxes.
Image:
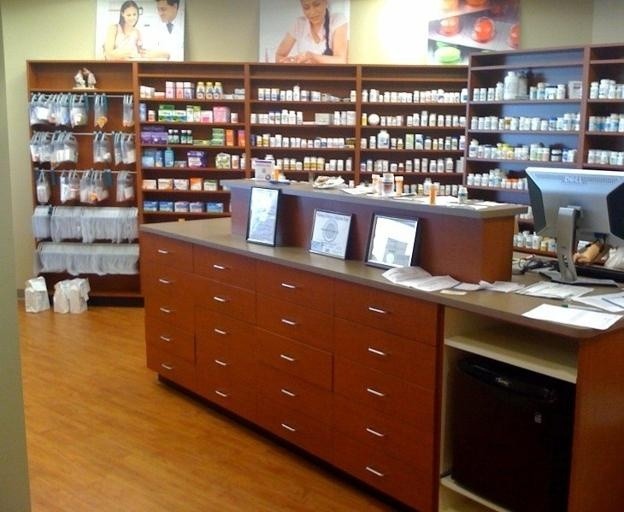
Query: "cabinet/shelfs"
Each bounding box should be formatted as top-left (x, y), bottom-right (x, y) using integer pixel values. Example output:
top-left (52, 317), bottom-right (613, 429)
top-left (136, 227), bottom-right (445, 511)
top-left (22, 42), bottom-right (622, 298)
top-left (438, 305), bottom-right (622, 507)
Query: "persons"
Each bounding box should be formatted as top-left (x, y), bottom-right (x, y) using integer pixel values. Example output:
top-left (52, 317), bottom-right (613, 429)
top-left (273, 0), bottom-right (348, 65)
top-left (103, 0), bottom-right (183, 62)
top-left (573, 241), bottom-right (623, 270)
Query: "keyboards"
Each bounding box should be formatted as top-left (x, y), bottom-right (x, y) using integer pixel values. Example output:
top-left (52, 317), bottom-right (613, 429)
top-left (555, 262), bottom-right (624, 284)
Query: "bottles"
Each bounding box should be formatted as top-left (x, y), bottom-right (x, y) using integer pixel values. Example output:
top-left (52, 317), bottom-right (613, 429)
top-left (513, 229), bottom-right (558, 255)
top-left (430, 184), bottom-right (438, 205)
top-left (469, 71), bottom-right (623, 190)
top-left (458, 187), bottom-right (468, 204)
top-left (248, 78), bottom-right (465, 197)
top-left (168, 129), bottom-right (192, 144)
top-left (197, 81), bottom-right (221, 99)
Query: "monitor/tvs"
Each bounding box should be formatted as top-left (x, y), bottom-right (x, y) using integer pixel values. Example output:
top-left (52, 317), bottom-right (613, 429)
top-left (525, 167), bottom-right (624, 288)
